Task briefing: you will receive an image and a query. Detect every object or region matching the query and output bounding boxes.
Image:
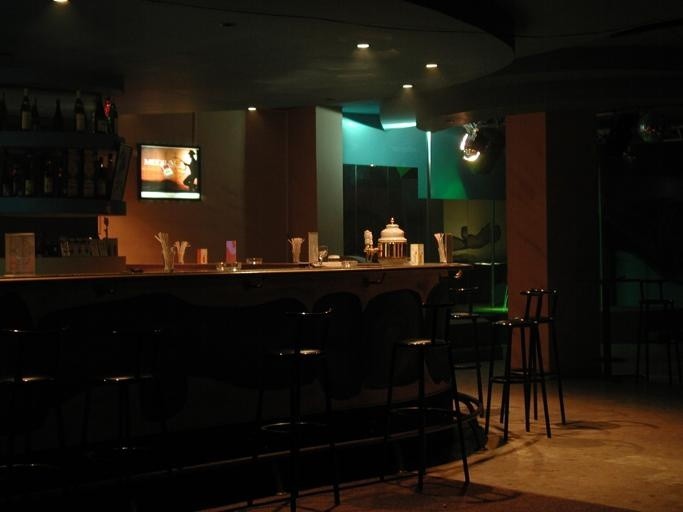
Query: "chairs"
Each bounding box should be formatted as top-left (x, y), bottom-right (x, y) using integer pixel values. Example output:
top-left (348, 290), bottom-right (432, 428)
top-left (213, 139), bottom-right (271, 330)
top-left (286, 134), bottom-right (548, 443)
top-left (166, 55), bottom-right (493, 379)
top-left (499, 285), bottom-right (568, 427)
top-left (382, 299), bottom-right (471, 493)
top-left (634, 280), bottom-right (677, 386)
top-left (448, 284), bottom-right (486, 419)
top-left (0, 318), bottom-right (77, 511)
top-left (482, 291), bottom-right (551, 442)
top-left (255, 307), bottom-right (342, 510)
top-left (78, 325), bottom-right (167, 512)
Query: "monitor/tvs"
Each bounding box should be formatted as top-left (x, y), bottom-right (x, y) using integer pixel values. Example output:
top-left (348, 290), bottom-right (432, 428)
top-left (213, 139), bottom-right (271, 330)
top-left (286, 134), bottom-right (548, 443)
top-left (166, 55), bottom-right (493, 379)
top-left (136, 142), bottom-right (202, 201)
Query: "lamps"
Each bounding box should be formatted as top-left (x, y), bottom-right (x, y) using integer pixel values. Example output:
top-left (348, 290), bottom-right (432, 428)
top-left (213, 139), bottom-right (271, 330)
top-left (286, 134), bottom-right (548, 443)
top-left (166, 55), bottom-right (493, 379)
top-left (452, 123), bottom-right (499, 170)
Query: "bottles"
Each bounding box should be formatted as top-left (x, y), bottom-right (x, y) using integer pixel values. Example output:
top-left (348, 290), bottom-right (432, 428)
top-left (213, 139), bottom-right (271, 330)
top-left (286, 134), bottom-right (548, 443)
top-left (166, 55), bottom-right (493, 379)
top-left (21, 86), bottom-right (32, 131)
top-left (108, 99), bottom-right (119, 136)
top-left (93, 92), bottom-right (105, 133)
top-left (0, 147), bottom-right (113, 198)
top-left (52, 99), bottom-right (65, 132)
top-left (72, 88), bottom-right (87, 132)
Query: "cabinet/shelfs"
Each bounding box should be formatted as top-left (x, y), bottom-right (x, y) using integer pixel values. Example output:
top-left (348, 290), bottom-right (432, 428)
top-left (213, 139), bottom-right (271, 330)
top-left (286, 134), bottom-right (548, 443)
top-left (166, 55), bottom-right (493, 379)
top-left (0, 123), bottom-right (128, 273)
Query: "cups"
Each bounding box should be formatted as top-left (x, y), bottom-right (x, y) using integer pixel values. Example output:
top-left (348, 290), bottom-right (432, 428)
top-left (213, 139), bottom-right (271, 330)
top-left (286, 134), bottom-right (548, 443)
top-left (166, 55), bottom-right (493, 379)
top-left (161, 244), bottom-right (177, 274)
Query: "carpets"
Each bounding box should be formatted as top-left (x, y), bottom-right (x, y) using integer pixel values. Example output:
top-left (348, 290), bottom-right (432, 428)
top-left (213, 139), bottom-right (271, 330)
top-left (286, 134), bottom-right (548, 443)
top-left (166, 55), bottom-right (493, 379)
top-left (199, 473), bottom-right (638, 511)
